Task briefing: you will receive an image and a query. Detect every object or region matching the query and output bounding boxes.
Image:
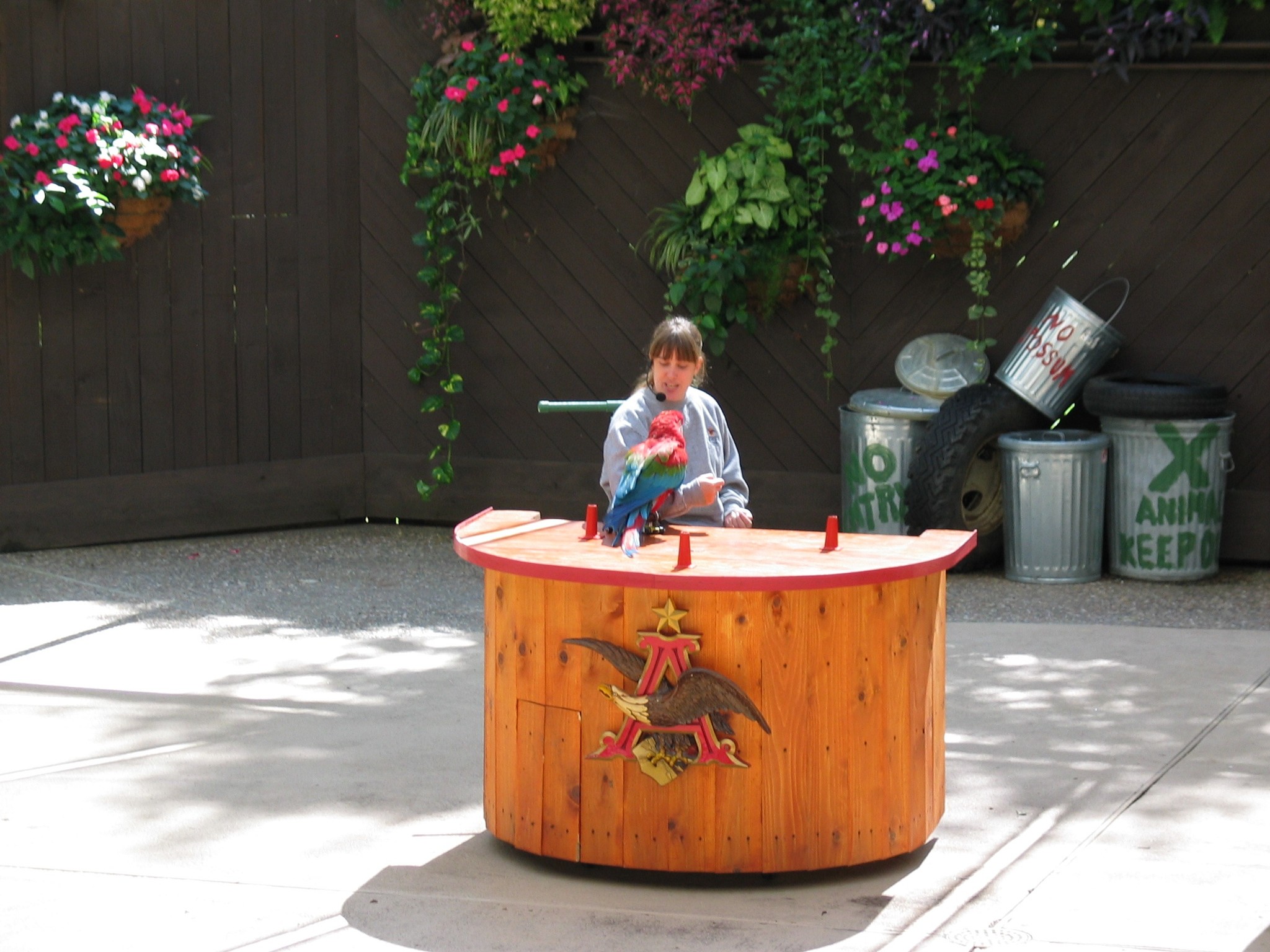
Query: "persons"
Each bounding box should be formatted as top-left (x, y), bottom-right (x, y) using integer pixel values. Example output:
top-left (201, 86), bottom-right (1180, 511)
top-left (600, 315), bottom-right (753, 529)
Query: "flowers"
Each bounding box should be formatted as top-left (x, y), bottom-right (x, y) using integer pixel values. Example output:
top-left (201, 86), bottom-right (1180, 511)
top-left (0, 85), bottom-right (212, 275)
top-left (440, 32), bottom-right (574, 197)
top-left (844, 117), bottom-right (991, 261)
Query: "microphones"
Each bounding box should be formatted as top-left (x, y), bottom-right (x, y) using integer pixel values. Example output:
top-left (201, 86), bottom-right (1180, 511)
top-left (646, 360), bottom-right (666, 402)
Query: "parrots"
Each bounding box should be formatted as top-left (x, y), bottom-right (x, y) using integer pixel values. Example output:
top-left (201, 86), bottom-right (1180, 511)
top-left (602, 410), bottom-right (689, 558)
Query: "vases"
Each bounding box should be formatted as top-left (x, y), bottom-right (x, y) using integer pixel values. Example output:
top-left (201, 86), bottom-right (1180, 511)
top-left (710, 244), bottom-right (819, 309)
top-left (94, 182), bottom-right (173, 254)
top-left (921, 199), bottom-right (1030, 262)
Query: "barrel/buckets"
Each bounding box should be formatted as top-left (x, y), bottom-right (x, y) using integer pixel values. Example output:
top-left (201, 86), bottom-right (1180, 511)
top-left (839, 404), bottom-right (932, 536)
top-left (994, 277), bottom-right (1130, 419)
top-left (1101, 406), bottom-right (1237, 579)
top-left (998, 429), bottom-right (1111, 583)
